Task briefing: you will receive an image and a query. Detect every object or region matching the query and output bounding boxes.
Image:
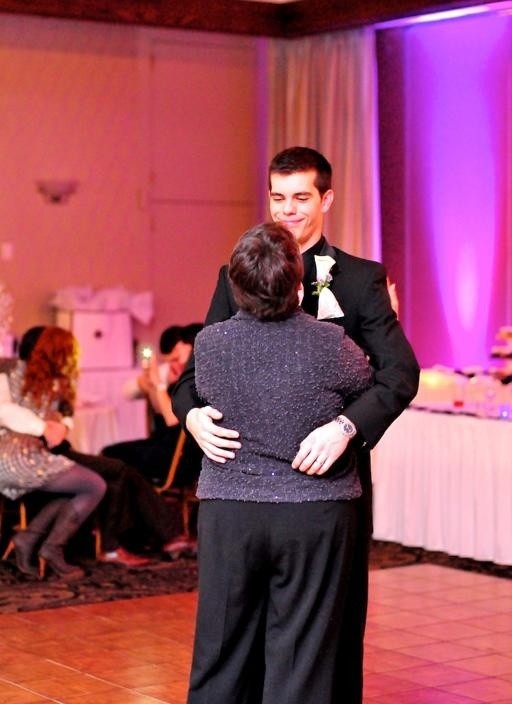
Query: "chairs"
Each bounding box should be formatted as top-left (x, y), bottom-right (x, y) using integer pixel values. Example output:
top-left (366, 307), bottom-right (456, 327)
top-left (156, 419), bottom-right (197, 556)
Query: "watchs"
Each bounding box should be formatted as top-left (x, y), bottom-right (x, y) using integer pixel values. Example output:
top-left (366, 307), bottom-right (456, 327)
top-left (334, 416), bottom-right (357, 437)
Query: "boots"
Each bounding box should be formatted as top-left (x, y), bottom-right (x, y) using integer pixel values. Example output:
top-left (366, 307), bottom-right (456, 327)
top-left (2, 496), bottom-right (86, 583)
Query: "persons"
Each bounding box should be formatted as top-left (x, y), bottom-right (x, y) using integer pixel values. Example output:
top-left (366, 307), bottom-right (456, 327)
top-left (0, 327), bottom-right (199, 571)
top-left (0, 327), bottom-right (109, 585)
top-left (169, 145), bottom-right (421, 704)
top-left (188, 220), bottom-right (400, 704)
top-left (103, 330), bottom-right (203, 485)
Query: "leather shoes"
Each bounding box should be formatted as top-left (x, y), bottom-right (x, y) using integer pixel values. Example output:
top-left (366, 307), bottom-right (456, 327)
top-left (163, 533), bottom-right (197, 561)
top-left (96, 546), bottom-right (160, 570)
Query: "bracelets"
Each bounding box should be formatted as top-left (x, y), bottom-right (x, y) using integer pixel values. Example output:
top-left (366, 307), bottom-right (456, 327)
top-left (155, 384), bottom-right (167, 390)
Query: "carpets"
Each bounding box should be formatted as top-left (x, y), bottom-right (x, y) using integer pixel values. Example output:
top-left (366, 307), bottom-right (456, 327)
top-left (0, 529), bottom-right (512, 613)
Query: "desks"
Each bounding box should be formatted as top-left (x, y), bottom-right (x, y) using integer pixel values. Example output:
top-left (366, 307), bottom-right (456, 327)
top-left (68, 392), bottom-right (154, 456)
top-left (368, 360), bottom-right (512, 565)
